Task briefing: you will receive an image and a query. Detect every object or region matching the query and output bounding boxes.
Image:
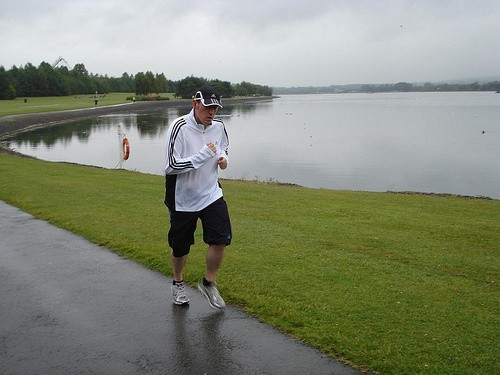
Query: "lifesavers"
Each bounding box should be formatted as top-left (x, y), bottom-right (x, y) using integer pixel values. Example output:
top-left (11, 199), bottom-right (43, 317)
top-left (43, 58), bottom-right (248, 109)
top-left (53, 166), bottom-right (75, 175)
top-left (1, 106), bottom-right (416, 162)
top-left (123, 138), bottom-right (130, 160)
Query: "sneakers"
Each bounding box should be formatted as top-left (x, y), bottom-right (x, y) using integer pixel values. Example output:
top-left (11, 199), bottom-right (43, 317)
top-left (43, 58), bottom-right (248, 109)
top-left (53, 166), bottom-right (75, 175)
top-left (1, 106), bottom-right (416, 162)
top-left (172, 284), bottom-right (190, 305)
top-left (199, 282), bottom-right (226, 311)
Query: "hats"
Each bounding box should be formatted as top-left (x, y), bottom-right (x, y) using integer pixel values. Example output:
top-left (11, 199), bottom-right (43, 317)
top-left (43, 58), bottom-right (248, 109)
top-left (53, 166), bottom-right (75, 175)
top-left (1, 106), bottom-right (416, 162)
top-left (193, 88), bottom-right (224, 108)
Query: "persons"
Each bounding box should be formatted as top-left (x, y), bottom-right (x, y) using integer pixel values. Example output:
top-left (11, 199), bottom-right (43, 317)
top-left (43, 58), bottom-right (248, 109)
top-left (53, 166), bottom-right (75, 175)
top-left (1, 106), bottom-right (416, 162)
top-left (94, 98), bottom-right (98, 107)
top-left (133, 96), bottom-right (135, 103)
top-left (164, 86), bottom-right (232, 308)
